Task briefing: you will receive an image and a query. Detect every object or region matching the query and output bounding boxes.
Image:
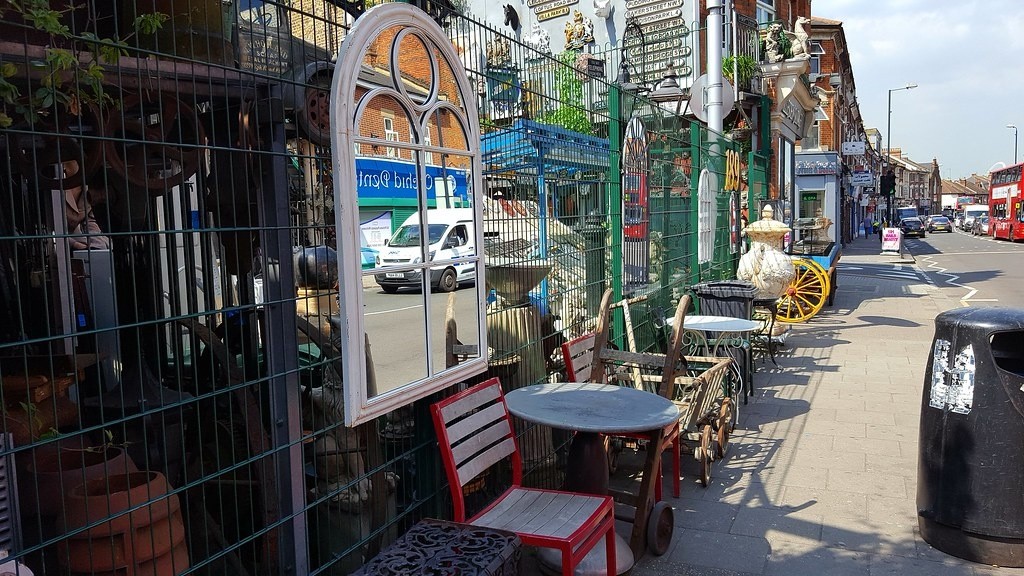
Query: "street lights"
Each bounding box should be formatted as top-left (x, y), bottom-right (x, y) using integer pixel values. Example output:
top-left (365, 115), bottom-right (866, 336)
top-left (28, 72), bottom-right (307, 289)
top-left (886, 84), bottom-right (919, 228)
top-left (1006, 125), bottom-right (1018, 165)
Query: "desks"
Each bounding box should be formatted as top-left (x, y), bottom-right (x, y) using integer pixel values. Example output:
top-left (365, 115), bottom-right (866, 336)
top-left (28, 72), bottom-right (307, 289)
top-left (346, 517), bottom-right (525, 576)
top-left (751, 298), bottom-right (784, 371)
top-left (504, 383), bottom-right (680, 576)
top-left (666, 315), bottom-right (760, 424)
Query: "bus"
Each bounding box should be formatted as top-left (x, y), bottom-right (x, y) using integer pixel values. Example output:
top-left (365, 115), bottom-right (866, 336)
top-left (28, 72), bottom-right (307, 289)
top-left (986, 161), bottom-right (1024, 244)
top-left (623, 172), bottom-right (647, 241)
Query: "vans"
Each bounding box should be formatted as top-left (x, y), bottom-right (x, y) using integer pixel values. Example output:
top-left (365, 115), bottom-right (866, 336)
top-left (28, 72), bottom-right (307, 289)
top-left (373, 207), bottom-right (476, 294)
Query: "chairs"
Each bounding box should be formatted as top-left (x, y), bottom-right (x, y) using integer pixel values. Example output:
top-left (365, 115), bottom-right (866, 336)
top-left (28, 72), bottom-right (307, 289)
top-left (670, 294), bottom-right (754, 405)
top-left (429, 376), bottom-right (617, 576)
top-left (562, 333), bottom-right (680, 503)
top-left (645, 306), bottom-right (733, 433)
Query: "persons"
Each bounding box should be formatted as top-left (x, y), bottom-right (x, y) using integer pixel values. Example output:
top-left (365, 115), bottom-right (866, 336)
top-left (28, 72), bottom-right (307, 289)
top-left (62, 160), bottom-right (107, 251)
top-left (881, 214), bottom-right (887, 224)
top-left (862, 213), bottom-right (872, 240)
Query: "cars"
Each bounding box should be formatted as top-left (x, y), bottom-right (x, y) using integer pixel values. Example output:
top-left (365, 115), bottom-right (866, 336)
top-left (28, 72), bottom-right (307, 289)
top-left (927, 216), bottom-right (952, 233)
top-left (896, 206), bottom-right (942, 238)
top-left (970, 213), bottom-right (990, 237)
top-left (953, 205), bottom-right (989, 233)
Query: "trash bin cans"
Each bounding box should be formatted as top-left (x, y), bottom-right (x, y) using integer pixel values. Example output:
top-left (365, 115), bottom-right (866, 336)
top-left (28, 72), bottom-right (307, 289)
top-left (916, 306), bottom-right (1024, 568)
top-left (689, 281), bottom-right (760, 388)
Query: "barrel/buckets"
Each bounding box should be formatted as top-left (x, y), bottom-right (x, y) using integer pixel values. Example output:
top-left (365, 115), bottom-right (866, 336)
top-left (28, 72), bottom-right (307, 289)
top-left (25, 446), bottom-right (141, 567)
top-left (54, 471), bottom-right (193, 576)
